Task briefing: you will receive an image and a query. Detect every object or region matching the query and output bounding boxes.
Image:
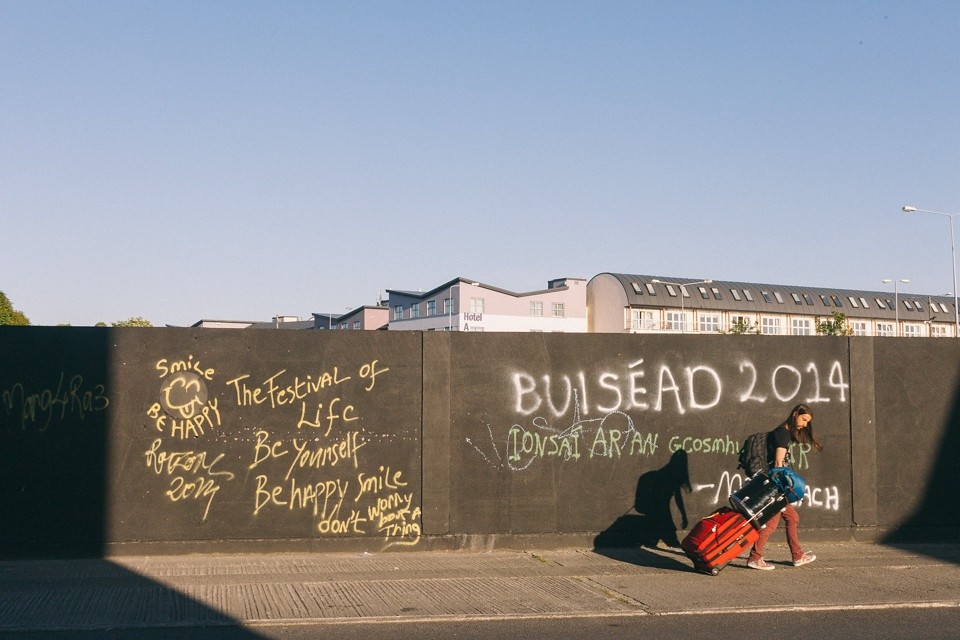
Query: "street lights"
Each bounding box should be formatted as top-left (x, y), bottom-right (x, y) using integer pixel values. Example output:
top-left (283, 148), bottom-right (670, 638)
top-left (928, 292), bottom-right (952, 338)
top-left (882, 279), bottom-right (910, 337)
top-left (650, 279), bottom-right (713, 334)
top-left (449, 283), bottom-right (479, 332)
top-left (902, 206), bottom-right (960, 337)
top-left (329, 307), bottom-right (352, 330)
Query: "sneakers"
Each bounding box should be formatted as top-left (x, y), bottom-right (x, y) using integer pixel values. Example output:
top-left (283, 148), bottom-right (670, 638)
top-left (794, 553), bottom-right (816, 567)
top-left (747, 556), bottom-right (775, 571)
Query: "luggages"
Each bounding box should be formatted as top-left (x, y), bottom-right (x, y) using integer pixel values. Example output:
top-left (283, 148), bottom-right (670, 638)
top-left (678, 506), bottom-right (761, 576)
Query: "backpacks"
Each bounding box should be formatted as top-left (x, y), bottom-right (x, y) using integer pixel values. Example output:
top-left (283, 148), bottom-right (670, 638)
top-left (737, 432), bottom-right (767, 476)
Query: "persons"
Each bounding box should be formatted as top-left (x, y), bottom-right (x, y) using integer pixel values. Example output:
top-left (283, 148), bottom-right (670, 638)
top-left (747, 404), bottom-right (823, 571)
